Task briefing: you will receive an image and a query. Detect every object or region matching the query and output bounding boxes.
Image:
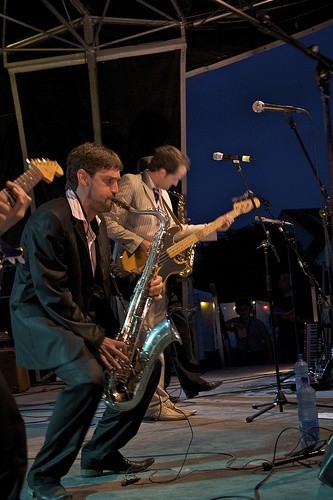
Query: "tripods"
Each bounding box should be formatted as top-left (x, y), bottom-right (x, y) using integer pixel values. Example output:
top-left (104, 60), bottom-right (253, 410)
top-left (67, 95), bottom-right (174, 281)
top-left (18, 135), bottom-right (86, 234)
top-left (246, 240), bottom-right (333, 423)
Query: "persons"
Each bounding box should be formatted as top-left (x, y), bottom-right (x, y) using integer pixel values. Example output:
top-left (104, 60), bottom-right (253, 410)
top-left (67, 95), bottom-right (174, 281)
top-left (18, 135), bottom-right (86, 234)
top-left (0, 180), bottom-right (27, 500)
top-left (222, 298), bottom-right (274, 360)
top-left (269, 303), bottom-right (304, 364)
top-left (10, 143), bottom-right (164, 500)
top-left (101, 144), bottom-right (235, 421)
top-left (137, 155), bottom-right (224, 403)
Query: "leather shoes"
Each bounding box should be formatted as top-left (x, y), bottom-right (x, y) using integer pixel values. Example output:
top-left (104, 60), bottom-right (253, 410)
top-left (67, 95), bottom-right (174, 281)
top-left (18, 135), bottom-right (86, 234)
top-left (185, 380), bottom-right (223, 398)
top-left (80, 451), bottom-right (155, 476)
top-left (27, 481), bottom-right (73, 500)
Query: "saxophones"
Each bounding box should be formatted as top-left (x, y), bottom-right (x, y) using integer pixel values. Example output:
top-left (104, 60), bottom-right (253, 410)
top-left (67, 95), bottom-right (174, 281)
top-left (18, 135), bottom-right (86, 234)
top-left (102, 197), bottom-right (183, 412)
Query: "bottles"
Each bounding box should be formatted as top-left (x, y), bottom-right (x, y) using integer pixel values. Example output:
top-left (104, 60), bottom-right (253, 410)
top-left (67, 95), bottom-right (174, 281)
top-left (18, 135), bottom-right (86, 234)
top-left (297, 377), bottom-right (319, 447)
top-left (294, 354), bottom-right (308, 390)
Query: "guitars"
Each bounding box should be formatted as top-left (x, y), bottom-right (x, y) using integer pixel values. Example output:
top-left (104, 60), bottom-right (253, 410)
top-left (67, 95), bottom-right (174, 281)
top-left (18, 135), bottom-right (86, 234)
top-left (123, 198), bottom-right (260, 285)
top-left (0, 158), bottom-right (63, 214)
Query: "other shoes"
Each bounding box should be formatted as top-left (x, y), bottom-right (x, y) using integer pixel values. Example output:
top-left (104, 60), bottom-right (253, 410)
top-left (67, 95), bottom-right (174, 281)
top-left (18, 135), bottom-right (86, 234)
top-left (144, 399), bottom-right (197, 420)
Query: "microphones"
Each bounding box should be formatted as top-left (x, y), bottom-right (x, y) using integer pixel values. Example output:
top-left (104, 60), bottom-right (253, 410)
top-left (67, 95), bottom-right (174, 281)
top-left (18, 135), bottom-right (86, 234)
top-left (213, 151), bottom-right (258, 163)
top-left (243, 189), bottom-right (272, 208)
top-left (255, 216), bottom-right (293, 226)
top-left (252, 101), bottom-right (304, 113)
